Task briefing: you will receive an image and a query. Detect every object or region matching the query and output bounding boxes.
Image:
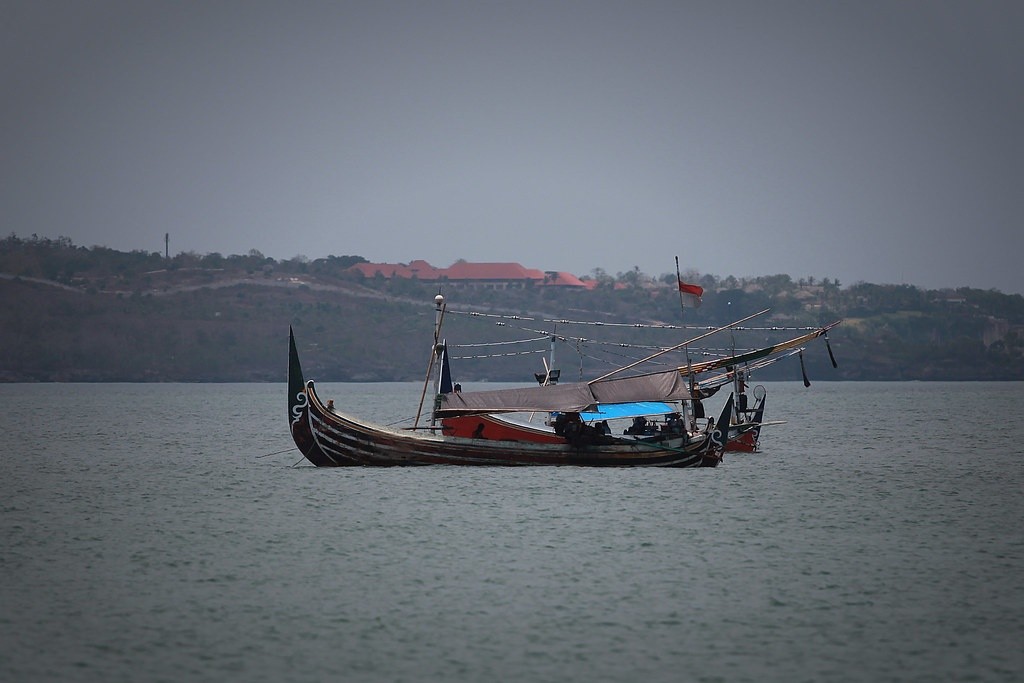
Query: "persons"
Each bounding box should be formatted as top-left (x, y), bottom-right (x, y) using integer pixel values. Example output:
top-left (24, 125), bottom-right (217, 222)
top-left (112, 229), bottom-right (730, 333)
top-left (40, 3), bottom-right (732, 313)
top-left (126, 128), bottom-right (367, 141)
top-left (624, 417), bottom-right (647, 435)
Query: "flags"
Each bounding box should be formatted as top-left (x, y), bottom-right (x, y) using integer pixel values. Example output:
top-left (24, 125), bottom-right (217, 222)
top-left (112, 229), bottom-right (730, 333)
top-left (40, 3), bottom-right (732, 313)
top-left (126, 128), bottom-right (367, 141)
top-left (679, 280), bottom-right (704, 298)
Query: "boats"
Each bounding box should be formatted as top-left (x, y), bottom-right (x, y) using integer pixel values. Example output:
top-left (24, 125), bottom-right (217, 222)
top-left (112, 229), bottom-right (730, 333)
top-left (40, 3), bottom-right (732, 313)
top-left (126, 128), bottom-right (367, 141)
top-left (427, 256), bottom-right (844, 454)
top-left (287, 322), bottom-right (736, 467)
